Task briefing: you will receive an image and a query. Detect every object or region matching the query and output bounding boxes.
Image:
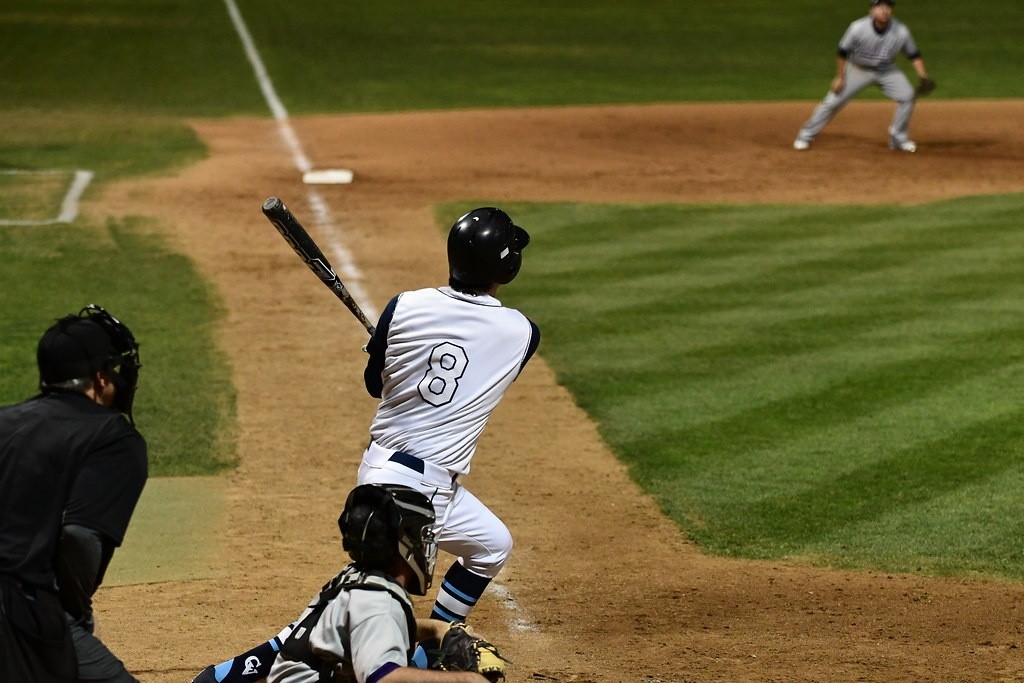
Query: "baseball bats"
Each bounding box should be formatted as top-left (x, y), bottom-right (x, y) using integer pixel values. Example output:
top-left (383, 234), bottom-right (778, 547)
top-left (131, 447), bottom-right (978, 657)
top-left (262, 196), bottom-right (375, 337)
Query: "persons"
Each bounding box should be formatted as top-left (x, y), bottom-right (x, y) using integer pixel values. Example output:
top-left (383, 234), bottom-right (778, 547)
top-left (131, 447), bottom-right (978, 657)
top-left (265, 482), bottom-right (493, 683)
top-left (187, 206), bottom-right (544, 683)
top-left (0, 303), bottom-right (151, 683)
top-left (793, 0), bottom-right (937, 154)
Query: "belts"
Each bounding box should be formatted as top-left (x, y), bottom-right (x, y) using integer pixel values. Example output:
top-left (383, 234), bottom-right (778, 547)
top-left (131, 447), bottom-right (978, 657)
top-left (362, 439), bottom-right (456, 490)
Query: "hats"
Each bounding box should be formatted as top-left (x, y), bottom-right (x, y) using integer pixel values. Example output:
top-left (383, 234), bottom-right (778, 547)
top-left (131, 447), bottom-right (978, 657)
top-left (871, 0), bottom-right (895, 7)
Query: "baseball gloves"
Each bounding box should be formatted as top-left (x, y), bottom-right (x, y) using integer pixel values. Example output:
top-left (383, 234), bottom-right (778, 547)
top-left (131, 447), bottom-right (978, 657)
top-left (440, 626), bottom-right (508, 682)
top-left (917, 75), bottom-right (934, 96)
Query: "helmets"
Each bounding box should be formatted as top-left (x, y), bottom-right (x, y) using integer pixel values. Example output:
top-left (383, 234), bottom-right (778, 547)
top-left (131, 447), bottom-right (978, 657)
top-left (336, 482), bottom-right (439, 595)
top-left (35, 303), bottom-right (140, 413)
top-left (448, 206), bottom-right (530, 288)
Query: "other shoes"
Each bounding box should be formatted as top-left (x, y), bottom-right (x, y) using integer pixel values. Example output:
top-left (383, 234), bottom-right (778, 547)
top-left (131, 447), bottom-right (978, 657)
top-left (793, 140), bottom-right (810, 150)
top-left (900, 140), bottom-right (919, 152)
